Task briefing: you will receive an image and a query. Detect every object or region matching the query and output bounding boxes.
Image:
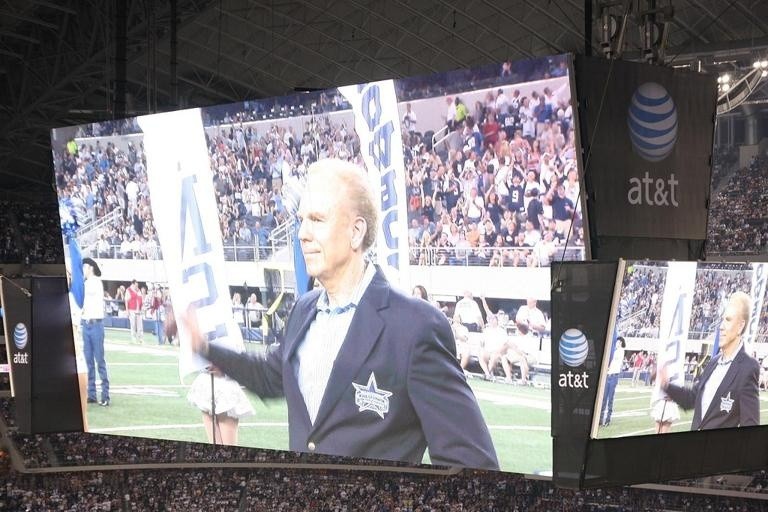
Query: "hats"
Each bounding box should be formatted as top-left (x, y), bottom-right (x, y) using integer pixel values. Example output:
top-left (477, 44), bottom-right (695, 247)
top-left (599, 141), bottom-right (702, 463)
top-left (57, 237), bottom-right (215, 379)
top-left (83, 257), bottom-right (102, 277)
top-left (617, 336), bottom-right (625, 348)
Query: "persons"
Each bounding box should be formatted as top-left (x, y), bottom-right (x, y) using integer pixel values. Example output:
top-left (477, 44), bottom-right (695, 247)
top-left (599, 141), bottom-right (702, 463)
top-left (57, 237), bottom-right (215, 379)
top-left (76, 257), bottom-right (111, 408)
top-left (1, 178), bottom-right (62, 278)
top-left (649, 370), bottom-right (679, 435)
top-left (163, 304), bottom-right (254, 447)
top-left (54, 57), bottom-right (587, 389)
top-left (0, 398), bottom-right (768, 512)
top-left (656, 290), bottom-right (762, 431)
top-left (703, 142), bottom-right (767, 258)
top-left (0, 374), bottom-right (10, 391)
top-left (596, 336), bottom-right (627, 426)
top-left (603, 261), bottom-right (768, 393)
top-left (180, 157), bottom-right (498, 470)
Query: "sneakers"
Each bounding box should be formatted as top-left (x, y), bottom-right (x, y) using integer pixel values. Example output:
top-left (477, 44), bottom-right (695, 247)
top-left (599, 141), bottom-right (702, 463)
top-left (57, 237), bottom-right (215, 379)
top-left (87, 398), bottom-right (108, 406)
